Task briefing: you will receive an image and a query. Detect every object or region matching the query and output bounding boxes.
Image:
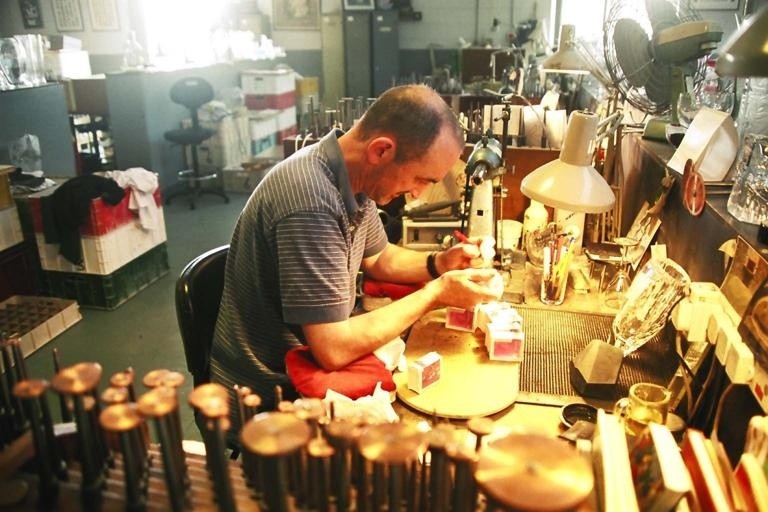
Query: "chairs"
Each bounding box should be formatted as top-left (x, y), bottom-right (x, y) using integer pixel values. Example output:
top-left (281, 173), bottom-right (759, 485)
top-left (164, 77), bottom-right (228, 210)
top-left (175, 241), bottom-right (239, 460)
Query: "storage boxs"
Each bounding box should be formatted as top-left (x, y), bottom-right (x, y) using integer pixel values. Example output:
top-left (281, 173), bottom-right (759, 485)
top-left (182, 70), bottom-right (319, 188)
top-left (0, 173), bottom-right (25, 252)
top-left (28, 178), bottom-right (167, 275)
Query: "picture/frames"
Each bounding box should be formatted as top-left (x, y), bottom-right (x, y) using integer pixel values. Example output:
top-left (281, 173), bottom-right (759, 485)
top-left (87, 0), bottom-right (121, 32)
top-left (272, 0), bottom-right (321, 31)
top-left (51, 0), bottom-right (84, 32)
top-left (342, 0), bottom-right (376, 11)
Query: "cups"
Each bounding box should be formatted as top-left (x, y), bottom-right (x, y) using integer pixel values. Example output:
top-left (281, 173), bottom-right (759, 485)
top-left (607, 257), bottom-right (691, 357)
top-left (495, 219), bottom-right (523, 252)
top-left (726, 131), bottom-right (768, 225)
top-left (538, 246), bottom-right (574, 304)
top-left (612, 382), bottom-right (672, 441)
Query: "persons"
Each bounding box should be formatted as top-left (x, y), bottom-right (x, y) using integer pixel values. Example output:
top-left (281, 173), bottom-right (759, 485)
top-left (210, 83), bottom-right (507, 446)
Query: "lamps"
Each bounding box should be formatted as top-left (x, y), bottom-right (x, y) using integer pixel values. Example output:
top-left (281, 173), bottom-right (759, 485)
top-left (537, 24), bottom-right (619, 116)
top-left (715, 3), bottom-right (767, 77)
top-left (519, 108), bottom-right (624, 214)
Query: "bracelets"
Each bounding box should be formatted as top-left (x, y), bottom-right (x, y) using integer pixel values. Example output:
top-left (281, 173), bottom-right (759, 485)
top-left (427, 247), bottom-right (443, 278)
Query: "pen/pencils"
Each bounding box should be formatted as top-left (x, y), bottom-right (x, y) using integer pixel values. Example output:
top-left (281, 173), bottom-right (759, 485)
top-left (454, 230), bottom-right (484, 263)
top-left (550, 235), bottom-right (581, 301)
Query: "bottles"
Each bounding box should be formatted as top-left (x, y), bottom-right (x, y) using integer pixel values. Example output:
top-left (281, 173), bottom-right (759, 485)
top-left (522, 198), bottom-right (550, 232)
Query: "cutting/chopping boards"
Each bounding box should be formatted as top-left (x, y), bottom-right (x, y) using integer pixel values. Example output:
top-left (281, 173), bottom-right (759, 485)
top-left (393, 308), bottom-right (520, 420)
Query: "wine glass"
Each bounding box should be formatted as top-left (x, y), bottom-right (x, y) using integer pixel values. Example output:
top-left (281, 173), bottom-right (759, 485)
top-left (604, 234), bottom-right (639, 311)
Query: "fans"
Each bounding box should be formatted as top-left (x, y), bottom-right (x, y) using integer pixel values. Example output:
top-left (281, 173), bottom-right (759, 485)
top-left (603, 0), bottom-right (724, 143)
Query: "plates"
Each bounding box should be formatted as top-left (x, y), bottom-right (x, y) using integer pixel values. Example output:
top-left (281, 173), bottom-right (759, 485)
top-left (561, 401), bottom-right (598, 432)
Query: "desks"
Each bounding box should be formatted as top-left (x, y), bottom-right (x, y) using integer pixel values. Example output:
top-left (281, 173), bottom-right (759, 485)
top-left (350, 235), bottom-right (705, 448)
